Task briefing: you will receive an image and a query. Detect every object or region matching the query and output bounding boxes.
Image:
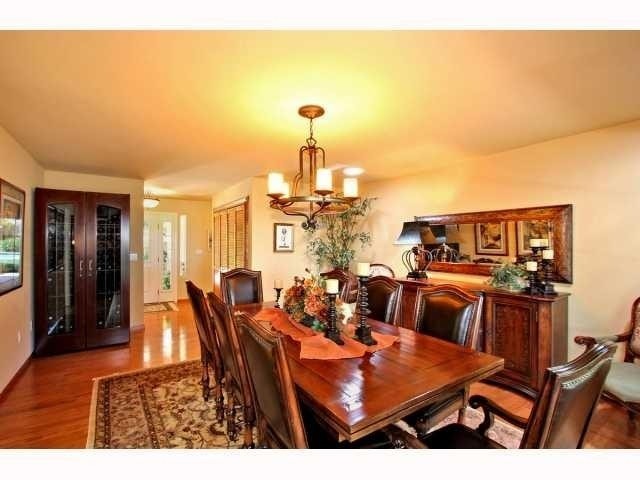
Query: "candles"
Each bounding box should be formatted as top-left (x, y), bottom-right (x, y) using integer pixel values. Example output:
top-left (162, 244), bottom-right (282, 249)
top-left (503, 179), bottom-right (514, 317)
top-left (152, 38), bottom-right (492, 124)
top-left (325, 279), bottom-right (339, 294)
top-left (529, 239), bottom-right (540, 247)
top-left (543, 249), bottom-right (553, 260)
top-left (274, 279), bottom-right (284, 288)
top-left (540, 239), bottom-right (548, 247)
top-left (357, 262), bottom-right (371, 276)
top-left (526, 261), bottom-right (537, 272)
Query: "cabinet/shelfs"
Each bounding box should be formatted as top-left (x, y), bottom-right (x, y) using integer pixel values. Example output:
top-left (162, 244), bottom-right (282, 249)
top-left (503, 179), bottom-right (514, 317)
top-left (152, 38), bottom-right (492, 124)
top-left (35, 188), bottom-right (131, 355)
top-left (394, 277), bottom-right (571, 400)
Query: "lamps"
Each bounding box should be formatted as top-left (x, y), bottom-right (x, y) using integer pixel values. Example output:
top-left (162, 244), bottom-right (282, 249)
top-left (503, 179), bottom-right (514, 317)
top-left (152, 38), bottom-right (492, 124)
top-left (429, 225), bottom-right (460, 262)
top-left (143, 191), bottom-right (160, 208)
top-left (267, 103), bottom-right (361, 234)
top-left (392, 221), bottom-right (438, 280)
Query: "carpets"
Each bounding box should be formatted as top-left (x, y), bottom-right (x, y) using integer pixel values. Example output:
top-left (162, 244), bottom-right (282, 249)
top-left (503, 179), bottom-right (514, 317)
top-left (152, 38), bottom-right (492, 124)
top-left (144, 302), bottom-right (179, 312)
top-left (86, 354), bottom-right (591, 448)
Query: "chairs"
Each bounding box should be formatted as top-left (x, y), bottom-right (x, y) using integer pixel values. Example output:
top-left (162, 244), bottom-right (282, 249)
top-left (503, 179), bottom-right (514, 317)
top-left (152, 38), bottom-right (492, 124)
top-left (574, 296), bottom-right (640, 421)
top-left (319, 270), bottom-right (351, 305)
top-left (206, 291), bottom-right (257, 449)
top-left (401, 284), bottom-right (483, 437)
top-left (359, 276), bottom-right (403, 327)
top-left (220, 267), bottom-right (263, 305)
top-left (230, 304), bottom-right (392, 449)
top-left (185, 281), bottom-right (225, 424)
top-left (394, 339), bottom-right (618, 449)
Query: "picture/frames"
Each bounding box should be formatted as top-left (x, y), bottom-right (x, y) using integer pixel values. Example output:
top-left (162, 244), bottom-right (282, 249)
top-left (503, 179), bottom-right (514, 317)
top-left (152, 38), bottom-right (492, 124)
top-left (274, 222), bottom-right (294, 252)
top-left (0, 177), bottom-right (26, 296)
top-left (475, 221), bottom-right (508, 255)
top-left (517, 219), bottom-right (553, 255)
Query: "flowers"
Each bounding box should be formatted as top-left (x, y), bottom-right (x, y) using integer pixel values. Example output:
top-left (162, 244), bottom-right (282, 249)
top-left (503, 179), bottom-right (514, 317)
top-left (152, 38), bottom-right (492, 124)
top-left (278, 268), bottom-right (356, 332)
top-left (484, 257), bottom-right (530, 292)
top-left (304, 193), bottom-right (376, 267)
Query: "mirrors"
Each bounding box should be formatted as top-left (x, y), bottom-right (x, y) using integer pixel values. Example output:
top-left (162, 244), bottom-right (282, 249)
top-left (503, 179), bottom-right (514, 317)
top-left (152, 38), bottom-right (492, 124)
top-left (414, 204), bottom-right (573, 284)
